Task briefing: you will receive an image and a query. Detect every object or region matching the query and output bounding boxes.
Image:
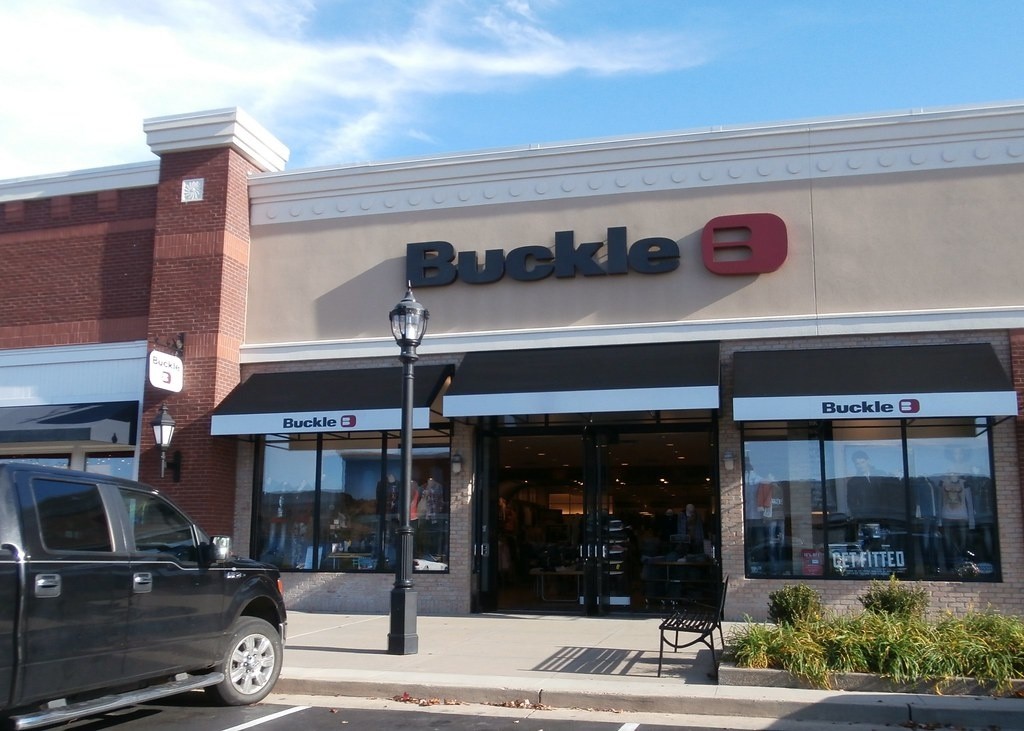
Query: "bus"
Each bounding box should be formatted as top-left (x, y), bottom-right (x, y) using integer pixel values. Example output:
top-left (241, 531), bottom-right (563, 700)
top-left (826, 544), bottom-right (862, 555)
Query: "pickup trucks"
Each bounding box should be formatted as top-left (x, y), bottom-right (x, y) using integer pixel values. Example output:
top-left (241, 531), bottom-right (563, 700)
top-left (0, 461), bottom-right (289, 731)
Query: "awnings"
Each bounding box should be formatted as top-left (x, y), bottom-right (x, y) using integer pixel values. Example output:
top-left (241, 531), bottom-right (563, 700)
top-left (443, 341), bottom-right (720, 418)
top-left (732, 343), bottom-right (1018, 421)
top-left (210, 363), bottom-right (454, 435)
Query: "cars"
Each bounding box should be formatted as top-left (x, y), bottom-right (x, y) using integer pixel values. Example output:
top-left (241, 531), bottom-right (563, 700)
top-left (413, 553), bottom-right (448, 572)
top-left (746, 542), bottom-right (835, 573)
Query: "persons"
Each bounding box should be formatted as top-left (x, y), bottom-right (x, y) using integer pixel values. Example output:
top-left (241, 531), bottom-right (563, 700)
top-left (916, 466), bottom-right (998, 572)
top-left (657, 504), bottom-right (704, 604)
top-left (264, 474), bottom-right (336, 568)
top-left (376, 474), bottom-right (443, 558)
top-left (847, 451), bottom-right (888, 526)
top-left (745, 470), bottom-right (785, 573)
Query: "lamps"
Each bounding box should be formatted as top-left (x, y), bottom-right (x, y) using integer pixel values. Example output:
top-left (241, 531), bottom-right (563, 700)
top-left (151, 404), bottom-right (184, 481)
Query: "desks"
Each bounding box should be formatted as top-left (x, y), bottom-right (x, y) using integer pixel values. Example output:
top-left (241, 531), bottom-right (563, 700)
top-left (649, 559), bottom-right (721, 594)
top-left (529, 566), bottom-right (584, 602)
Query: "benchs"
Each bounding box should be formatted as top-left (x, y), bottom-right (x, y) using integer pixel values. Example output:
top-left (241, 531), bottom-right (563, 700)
top-left (657, 572), bottom-right (729, 679)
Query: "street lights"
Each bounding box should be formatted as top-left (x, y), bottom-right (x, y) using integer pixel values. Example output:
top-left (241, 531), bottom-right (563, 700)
top-left (383, 275), bottom-right (430, 655)
